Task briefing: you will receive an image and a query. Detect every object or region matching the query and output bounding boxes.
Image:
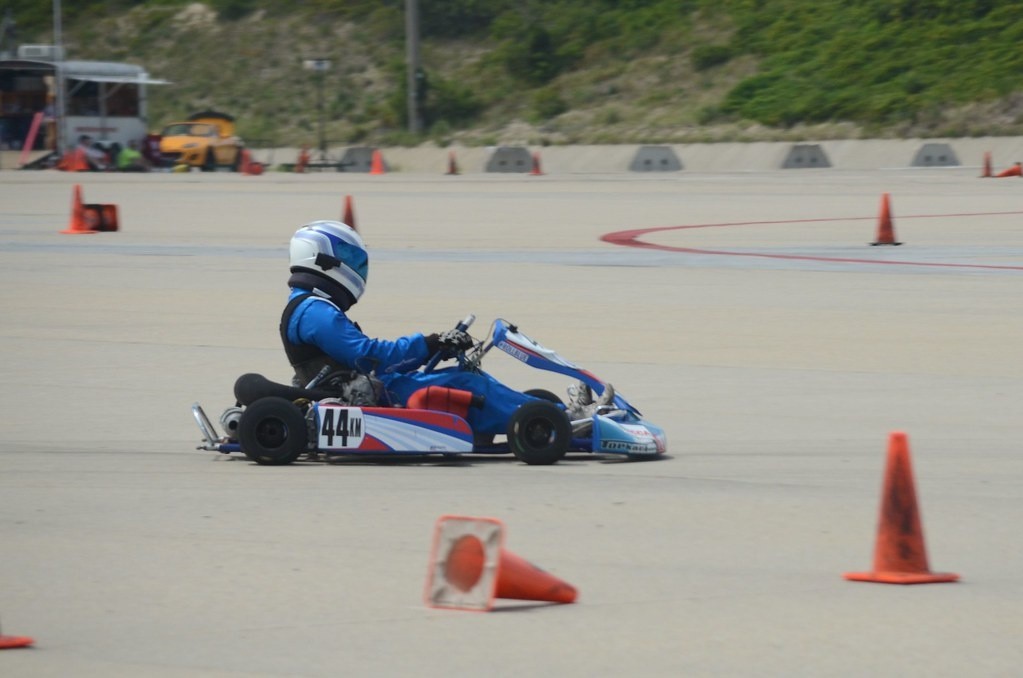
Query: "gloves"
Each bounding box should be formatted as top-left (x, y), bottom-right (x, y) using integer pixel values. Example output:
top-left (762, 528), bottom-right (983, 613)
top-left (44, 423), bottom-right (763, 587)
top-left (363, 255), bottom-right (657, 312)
top-left (424, 329), bottom-right (474, 362)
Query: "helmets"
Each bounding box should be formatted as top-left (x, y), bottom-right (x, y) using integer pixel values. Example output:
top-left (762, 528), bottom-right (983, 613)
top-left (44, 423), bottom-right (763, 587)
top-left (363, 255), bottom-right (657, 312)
top-left (286, 220), bottom-right (370, 314)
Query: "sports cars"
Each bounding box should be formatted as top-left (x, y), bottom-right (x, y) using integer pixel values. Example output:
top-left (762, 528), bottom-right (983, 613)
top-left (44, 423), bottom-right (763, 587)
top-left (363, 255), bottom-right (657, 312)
top-left (153, 112), bottom-right (245, 175)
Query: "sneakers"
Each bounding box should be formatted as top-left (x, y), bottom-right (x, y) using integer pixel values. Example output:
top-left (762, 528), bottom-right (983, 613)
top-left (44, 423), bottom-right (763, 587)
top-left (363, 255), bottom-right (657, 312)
top-left (567, 381), bottom-right (592, 408)
top-left (565, 383), bottom-right (614, 423)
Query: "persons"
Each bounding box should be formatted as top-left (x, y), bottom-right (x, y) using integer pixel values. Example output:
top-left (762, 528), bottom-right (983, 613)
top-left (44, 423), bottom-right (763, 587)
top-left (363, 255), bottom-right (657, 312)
top-left (73, 134), bottom-right (109, 171)
top-left (279, 221), bottom-right (615, 436)
top-left (116, 139), bottom-right (161, 173)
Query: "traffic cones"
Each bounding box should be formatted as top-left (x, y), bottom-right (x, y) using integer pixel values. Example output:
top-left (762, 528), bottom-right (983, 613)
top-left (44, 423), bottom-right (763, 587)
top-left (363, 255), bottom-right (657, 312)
top-left (995, 163), bottom-right (1022, 178)
top-left (979, 153), bottom-right (999, 178)
top-left (59, 184), bottom-right (107, 236)
top-left (527, 151), bottom-right (544, 175)
top-left (292, 146), bottom-right (311, 172)
top-left (871, 191), bottom-right (907, 244)
top-left (341, 192), bottom-right (357, 230)
top-left (244, 162), bottom-right (263, 176)
top-left (0, 630), bottom-right (35, 650)
top-left (364, 151), bottom-right (384, 175)
top-left (425, 515), bottom-right (579, 612)
top-left (81, 201), bottom-right (121, 229)
top-left (839, 431), bottom-right (966, 587)
top-left (237, 152), bottom-right (250, 176)
top-left (446, 148), bottom-right (461, 177)
